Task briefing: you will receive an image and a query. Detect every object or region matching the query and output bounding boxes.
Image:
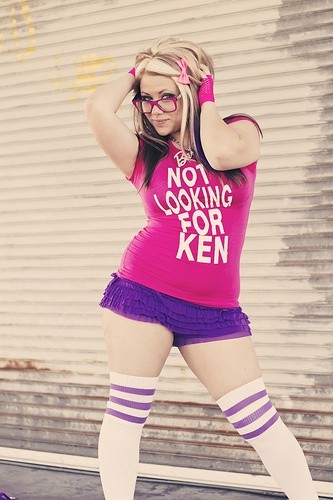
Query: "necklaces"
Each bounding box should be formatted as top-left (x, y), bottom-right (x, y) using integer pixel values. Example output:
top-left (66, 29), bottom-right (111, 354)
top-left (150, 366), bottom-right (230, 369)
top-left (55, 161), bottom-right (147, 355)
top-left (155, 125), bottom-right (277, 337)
top-left (168, 134), bottom-right (193, 152)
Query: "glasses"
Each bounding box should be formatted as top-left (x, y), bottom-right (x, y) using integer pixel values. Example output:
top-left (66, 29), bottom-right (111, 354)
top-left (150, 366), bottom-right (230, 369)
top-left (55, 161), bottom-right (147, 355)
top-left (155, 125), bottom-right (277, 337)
top-left (132, 92), bottom-right (182, 114)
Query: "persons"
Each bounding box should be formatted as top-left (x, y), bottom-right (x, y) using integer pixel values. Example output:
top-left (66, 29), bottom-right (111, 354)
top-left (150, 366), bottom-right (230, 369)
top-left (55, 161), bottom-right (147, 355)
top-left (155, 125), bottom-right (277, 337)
top-left (84, 38), bottom-right (319, 500)
